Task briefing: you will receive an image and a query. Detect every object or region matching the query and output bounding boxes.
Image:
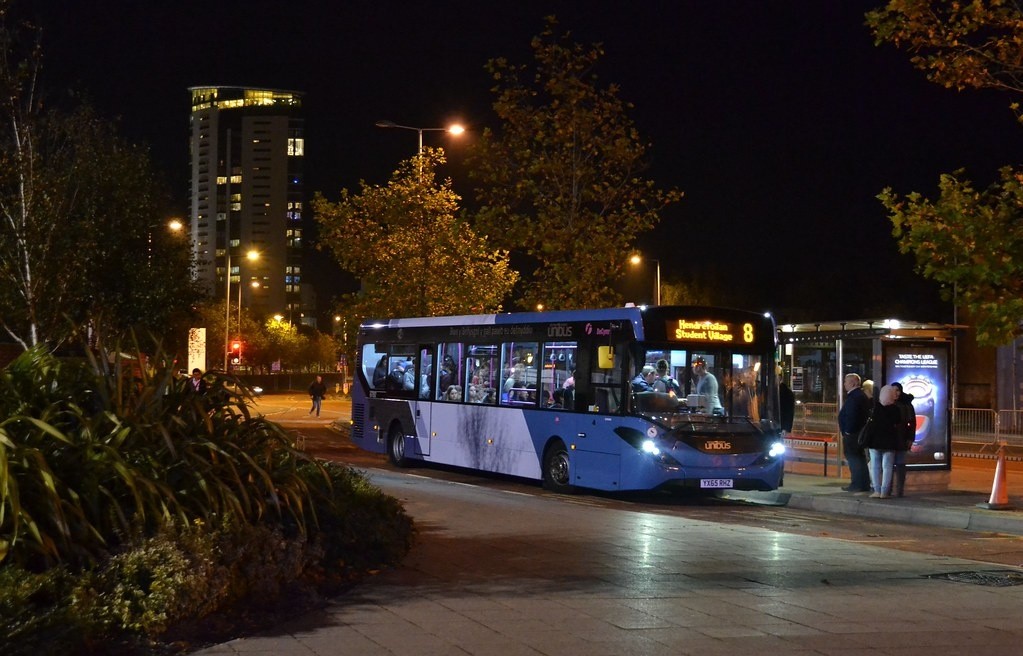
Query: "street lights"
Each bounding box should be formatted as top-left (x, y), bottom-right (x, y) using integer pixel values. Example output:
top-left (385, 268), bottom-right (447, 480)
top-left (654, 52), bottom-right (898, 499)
top-left (376, 120), bottom-right (466, 317)
top-left (224, 248), bottom-right (259, 371)
top-left (630, 255), bottom-right (660, 306)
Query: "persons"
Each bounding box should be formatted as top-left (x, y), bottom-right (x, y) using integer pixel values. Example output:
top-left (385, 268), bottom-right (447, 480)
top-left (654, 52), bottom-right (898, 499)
top-left (631, 366), bottom-right (657, 392)
top-left (439, 359), bottom-right (462, 401)
top-left (373, 355), bottom-right (429, 398)
top-left (467, 362), bottom-right (496, 403)
top-left (504, 363), bottom-right (536, 402)
top-left (693, 356), bottom-right (721, 408)
top-left (779, 373), bottom-right (796, 487)
top-left (728, 369), bottom-right (760, 416)
top-left (308, 375), bottom-right (326, 418)
top-left (653, 359), bottom-right (679, 393)
top-left (837, 373), bottom-right (916, 499)
top-left (552, 364), bottom-right (575, 408)
top-left (187, 369), bottom-right (206, 392)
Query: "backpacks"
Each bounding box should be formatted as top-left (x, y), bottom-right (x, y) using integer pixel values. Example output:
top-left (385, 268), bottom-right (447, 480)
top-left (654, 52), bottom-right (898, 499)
top-left (657, 377), bottom-right (683, 398)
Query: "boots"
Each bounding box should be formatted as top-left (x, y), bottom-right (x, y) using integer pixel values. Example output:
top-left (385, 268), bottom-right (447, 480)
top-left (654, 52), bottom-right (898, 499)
top-left (895, 463), bottom-right (905, 498)
top-left (889, 474), bottom-right (893, 496)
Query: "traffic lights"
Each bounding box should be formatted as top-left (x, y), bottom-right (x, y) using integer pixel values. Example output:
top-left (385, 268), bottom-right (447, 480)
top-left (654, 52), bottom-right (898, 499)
top-left (232, 341), bottom-right (240, 355)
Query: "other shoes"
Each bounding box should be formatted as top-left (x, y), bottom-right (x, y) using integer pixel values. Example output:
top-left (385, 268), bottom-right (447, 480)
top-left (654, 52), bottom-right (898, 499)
top-left (863, 485), bottom-right (870, 490)
top-left (316, 414), bottom-right (320, 418)
top-left (880, 494), bottom-right (887, 499)
top-left (308, 412), bottom-right (311, 416)
top-left (841, 484), bottom-right (862, 492)
top-left (869, 492), bottom-right (880, 498)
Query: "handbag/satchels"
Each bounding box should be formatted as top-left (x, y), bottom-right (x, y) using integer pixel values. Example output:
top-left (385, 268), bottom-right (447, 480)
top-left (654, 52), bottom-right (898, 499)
top-left (857, 420), bottom-right (872, 449)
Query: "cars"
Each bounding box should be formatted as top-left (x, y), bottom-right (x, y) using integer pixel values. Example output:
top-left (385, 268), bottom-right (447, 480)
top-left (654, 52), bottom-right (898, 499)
top-left (201, 369), bottom-right (263, 397)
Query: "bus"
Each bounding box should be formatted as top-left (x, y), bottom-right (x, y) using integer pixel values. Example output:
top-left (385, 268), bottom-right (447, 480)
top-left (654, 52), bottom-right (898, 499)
top-left (349, 304), bottom-right (787, 497)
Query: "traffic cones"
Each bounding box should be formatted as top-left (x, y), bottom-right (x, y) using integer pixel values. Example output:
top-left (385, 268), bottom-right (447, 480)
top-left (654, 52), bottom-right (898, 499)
top-left (975, 448), bottom-right (1017, 511)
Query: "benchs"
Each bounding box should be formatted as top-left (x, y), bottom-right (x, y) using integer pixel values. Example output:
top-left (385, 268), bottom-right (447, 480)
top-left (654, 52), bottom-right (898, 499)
top-left (782, 435), bottom-right (832, 477)
